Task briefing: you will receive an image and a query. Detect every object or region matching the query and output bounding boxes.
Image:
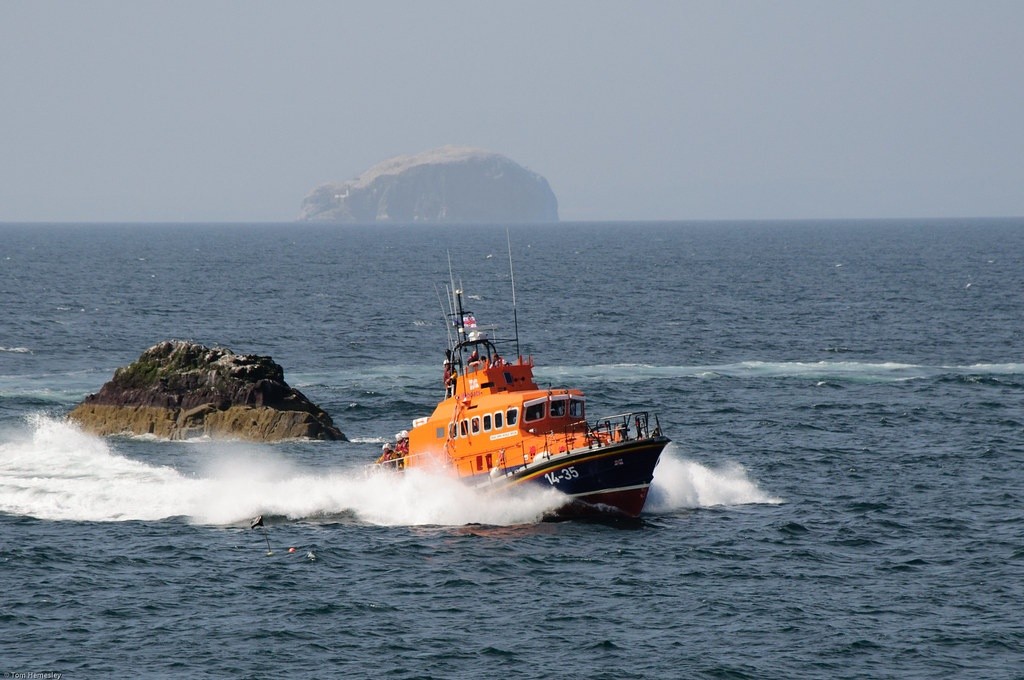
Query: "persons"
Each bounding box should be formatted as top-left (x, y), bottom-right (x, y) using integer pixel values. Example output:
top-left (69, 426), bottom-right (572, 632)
top-left (444, 351), bottom-right (503, 392)
top-left (376, 430), bottom-right (409, 468)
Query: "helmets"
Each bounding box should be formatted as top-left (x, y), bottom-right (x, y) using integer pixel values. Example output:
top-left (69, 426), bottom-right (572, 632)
top-left (443, 359), bottom-right (449, 365)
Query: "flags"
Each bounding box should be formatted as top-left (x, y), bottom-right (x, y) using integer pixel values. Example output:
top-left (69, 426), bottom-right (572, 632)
top-left (463, 317), bottom-right (476, 327)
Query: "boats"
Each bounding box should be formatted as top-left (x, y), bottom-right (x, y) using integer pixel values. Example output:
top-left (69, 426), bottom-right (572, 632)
top-left (364, 226), bottom-right (671, 522)
